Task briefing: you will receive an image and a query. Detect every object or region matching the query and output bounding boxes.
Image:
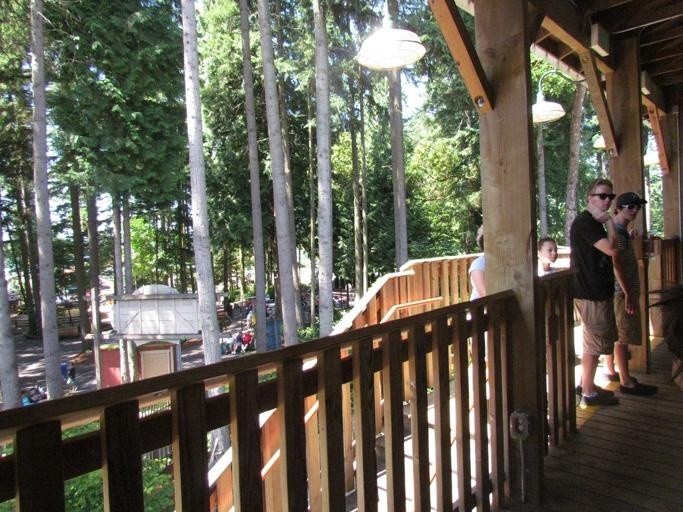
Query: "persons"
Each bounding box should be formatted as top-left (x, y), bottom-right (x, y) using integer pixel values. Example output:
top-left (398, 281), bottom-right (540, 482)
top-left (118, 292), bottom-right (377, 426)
top-left (230, 331), bottom-right (243, 355)
top-left (246, 305), bottom-right (254, 330)
top-left (67, 362), bottom-right (78, 392)
top-left (468, 221), bottom-right (490, 365)
top-left (240, 330), bottom-right (253, 353)
top-left (59, 359), bottom-right (69, 384)
top-left (602, 192), bottom-right (659, 396)
top-left (569, 178), bottom-right (618, 406)
top-left (536, 237), bottom-right (566, 277)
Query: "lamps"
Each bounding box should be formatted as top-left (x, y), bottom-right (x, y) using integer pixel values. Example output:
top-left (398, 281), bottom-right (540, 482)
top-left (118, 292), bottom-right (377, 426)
top-left (357, 1), bottom-right (426, 72)
top-left (529, 70), bottom-right (588, 123)
top-left (591, 129), bottom-right (607, 151)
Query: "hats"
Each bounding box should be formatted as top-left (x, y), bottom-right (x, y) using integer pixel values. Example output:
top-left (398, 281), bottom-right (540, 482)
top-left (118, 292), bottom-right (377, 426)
top-left (616, 192), bottom-right (648, 205)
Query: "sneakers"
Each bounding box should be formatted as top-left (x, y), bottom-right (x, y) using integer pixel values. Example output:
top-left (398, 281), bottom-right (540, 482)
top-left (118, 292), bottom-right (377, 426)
top-left (576, 385), bottom-right (619, 406)
top-left (619, 381), bottom-right (659, 397)
top-left (607, 371), bottom-right (638, 382)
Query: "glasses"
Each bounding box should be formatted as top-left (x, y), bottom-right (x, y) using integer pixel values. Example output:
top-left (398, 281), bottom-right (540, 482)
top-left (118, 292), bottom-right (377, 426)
top-left (588, 192), bottom-right (617, 201)
top-left (620, 204), bottom-right (642, 210)
